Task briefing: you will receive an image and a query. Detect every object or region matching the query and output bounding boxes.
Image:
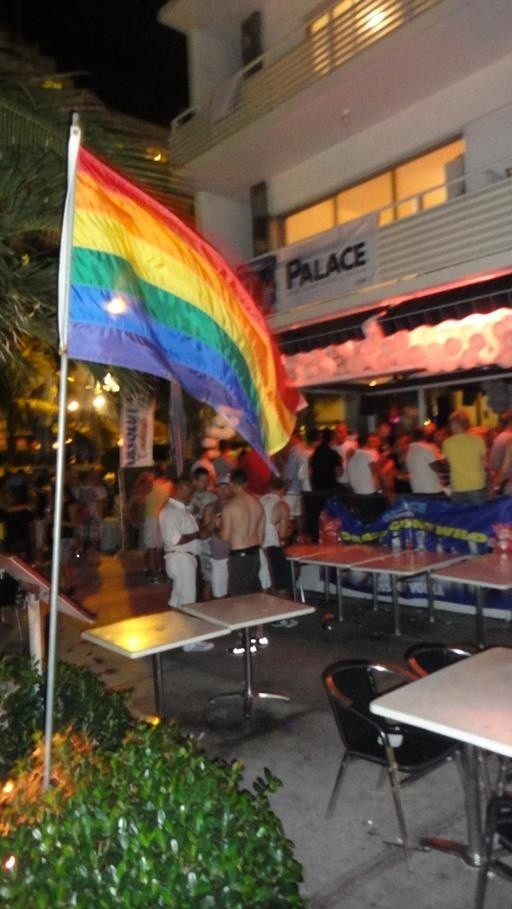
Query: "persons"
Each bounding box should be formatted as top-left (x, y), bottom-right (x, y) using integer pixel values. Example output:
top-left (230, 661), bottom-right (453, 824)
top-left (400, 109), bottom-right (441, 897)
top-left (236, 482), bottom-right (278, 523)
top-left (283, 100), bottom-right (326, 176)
top-left (69, 470), bottom-right (108, 568)
top-left (188, 465), bottom-right (218, 601)
top-left (193, 440), bottom-right (271, 495)
top-left (157, 478), bottom-right (215, 653)
top-left (38, 435), bottom-right (57, 464)
top-left (2, 464), bottom-right (115, 535)
top-left (280, 408), bottom-right (512, 535)
top-left (1, 484), bottom-right (32, 567)
top-left (257, 476), bottom-right (299, 629)
top-left (218, 468), bottom-right (266, 655)
top-left (202, 475), bottom-right (231, 600)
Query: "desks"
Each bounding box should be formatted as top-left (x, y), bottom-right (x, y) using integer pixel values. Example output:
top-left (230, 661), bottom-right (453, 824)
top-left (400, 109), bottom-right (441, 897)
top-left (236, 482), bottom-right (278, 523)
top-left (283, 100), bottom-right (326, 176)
top-left (282, 542), bottom-right (510, 648)
top-left (369, 648), bottom-right (511, 908)
top-left (78, 588), bottom-right (318, 750)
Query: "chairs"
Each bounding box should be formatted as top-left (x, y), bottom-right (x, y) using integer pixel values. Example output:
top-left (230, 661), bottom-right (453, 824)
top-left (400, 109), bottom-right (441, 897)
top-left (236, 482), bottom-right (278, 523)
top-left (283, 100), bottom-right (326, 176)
top-left (317, 638), bottom-right (470, 880)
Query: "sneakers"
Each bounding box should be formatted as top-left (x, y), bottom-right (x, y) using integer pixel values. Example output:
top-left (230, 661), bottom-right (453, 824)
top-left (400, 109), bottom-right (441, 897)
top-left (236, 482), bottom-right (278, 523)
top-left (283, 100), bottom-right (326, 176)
top-left (225, 636), bottom-right (268, 655)
top-left (182, 641), bottom-right (215, 653)
top-left (270, 620), bottom-right (299, 629)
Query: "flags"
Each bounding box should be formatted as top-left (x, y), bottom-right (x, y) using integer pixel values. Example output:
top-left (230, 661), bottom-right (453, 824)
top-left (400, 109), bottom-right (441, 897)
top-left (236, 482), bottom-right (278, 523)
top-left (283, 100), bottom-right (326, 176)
top-left (120, 397), bottom-right (156, 466)
top-left (58, 142), bottom-right (311, 464)
